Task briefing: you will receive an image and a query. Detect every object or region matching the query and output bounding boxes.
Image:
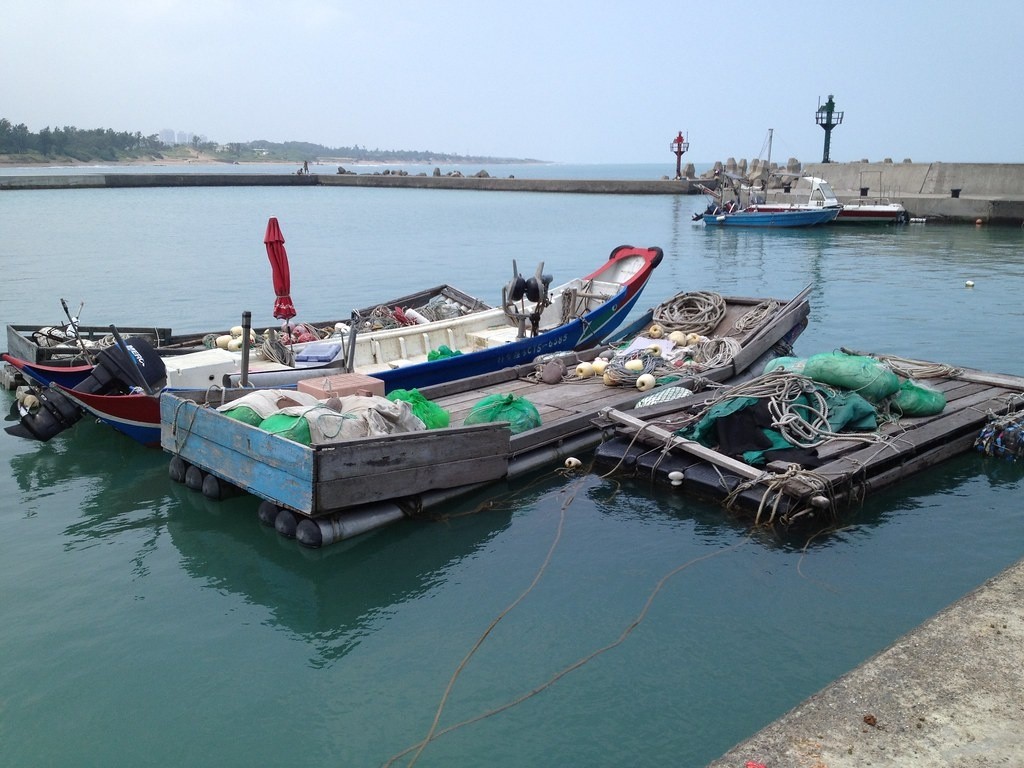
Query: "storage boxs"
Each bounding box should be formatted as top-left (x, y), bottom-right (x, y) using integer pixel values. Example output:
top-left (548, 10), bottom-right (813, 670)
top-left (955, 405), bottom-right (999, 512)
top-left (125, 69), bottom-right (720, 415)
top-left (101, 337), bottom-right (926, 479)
top-left (296, 372), bottom-right (385, 400)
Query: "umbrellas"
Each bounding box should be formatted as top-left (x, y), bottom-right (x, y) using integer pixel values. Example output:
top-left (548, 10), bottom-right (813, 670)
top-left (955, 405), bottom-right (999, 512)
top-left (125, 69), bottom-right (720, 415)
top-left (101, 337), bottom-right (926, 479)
top-left (263, 218), bottom-right (297, 344)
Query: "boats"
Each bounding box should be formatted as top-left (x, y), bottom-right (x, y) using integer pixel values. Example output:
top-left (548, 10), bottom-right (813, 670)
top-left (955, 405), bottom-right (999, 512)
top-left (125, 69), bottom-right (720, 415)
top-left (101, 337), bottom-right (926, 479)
top-left (0, 283), bottom-right (494, 392)
top-left (691, 129), bottom-right (905, 228)
top-left (160, 283), bottom-right (814, 549)
top-left (1, 215), bottom-right (664, 448)
top-left (590, 344), bottom-right (1024, 537)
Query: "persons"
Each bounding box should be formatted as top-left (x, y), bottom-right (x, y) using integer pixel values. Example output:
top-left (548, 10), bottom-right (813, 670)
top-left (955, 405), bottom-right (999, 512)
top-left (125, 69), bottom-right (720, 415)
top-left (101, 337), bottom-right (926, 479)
top-left (297, 160), bottom-right (309, 176)
